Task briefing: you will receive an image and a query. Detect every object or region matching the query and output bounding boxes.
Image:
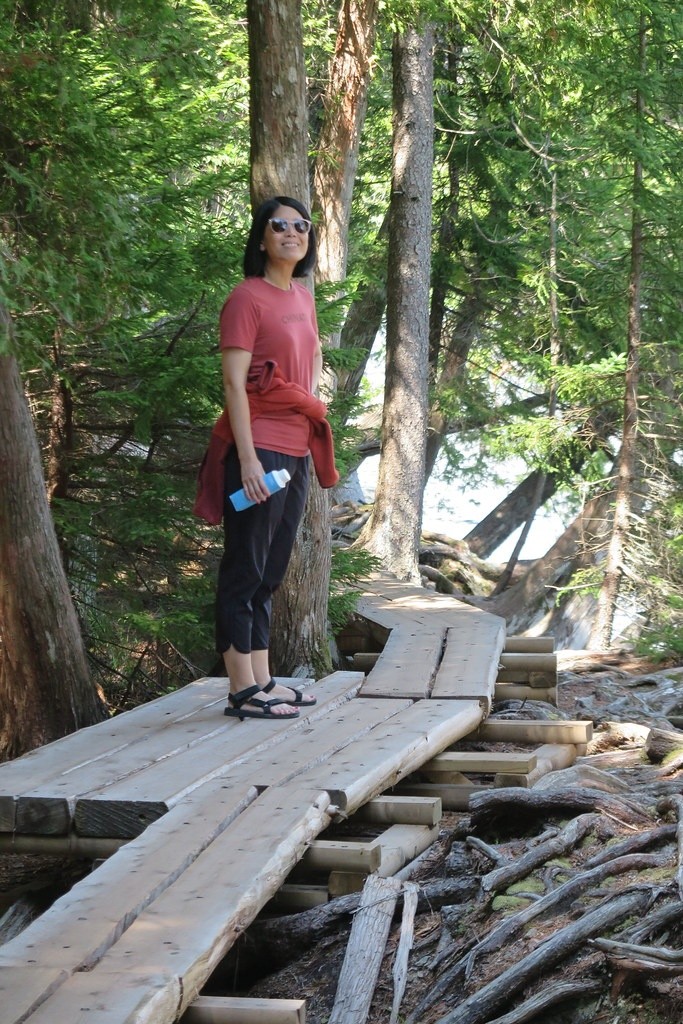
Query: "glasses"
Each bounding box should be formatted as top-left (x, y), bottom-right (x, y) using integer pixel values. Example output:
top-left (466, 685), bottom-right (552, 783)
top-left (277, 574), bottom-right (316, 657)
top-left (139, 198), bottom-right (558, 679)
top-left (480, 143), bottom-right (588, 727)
top-left (268, 217), bottom-right (312, 234)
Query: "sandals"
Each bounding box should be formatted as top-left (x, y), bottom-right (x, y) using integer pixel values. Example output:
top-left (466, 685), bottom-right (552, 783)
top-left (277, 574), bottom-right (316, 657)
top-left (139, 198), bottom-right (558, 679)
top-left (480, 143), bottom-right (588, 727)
top-left (259, 676), bottom-right (318, 706)
top-left (224, 684), bottom-right (301, 718)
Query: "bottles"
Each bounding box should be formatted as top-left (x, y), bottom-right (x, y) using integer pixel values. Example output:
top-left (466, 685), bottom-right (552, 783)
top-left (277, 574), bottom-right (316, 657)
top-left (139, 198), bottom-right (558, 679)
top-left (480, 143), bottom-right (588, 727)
top-left (229, 468), bottom-right (291, 511)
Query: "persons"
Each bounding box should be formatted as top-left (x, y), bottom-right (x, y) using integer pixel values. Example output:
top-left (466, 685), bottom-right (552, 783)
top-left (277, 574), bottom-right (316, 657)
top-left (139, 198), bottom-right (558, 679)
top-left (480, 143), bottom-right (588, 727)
top-left (192, 197), bottom-right (338, 717)
